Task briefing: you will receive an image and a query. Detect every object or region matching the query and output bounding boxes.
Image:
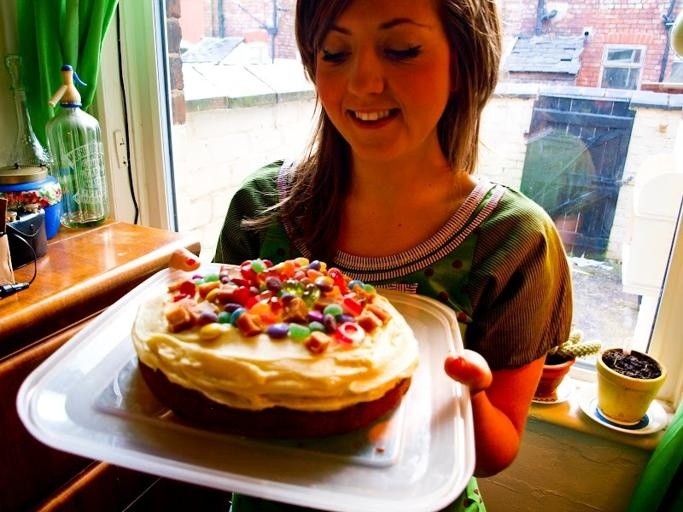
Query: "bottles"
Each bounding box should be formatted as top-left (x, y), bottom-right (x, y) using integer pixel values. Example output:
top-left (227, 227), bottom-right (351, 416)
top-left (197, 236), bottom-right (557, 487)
top-left (2, 57), bottom-right (48, 166)
top-left (42, 103), bottom-right (111, 230)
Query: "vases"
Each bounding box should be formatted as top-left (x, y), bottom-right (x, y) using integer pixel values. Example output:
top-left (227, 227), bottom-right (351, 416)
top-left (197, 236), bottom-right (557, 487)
top-left (599, 346), bottom-right (667, 428)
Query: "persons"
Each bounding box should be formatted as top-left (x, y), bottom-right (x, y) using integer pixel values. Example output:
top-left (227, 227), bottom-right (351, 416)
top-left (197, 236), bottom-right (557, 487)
top-left (163, 1), bottom-right (572, 511)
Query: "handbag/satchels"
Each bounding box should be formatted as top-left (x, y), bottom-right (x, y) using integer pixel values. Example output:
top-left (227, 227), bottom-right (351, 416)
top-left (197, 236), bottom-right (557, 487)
top-left (6, 209), bottom-right (47, 270)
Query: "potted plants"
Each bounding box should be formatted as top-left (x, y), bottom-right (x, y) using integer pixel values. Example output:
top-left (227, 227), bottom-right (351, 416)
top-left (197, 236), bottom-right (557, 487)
top-left (534, 331), bottom-right (601, 399)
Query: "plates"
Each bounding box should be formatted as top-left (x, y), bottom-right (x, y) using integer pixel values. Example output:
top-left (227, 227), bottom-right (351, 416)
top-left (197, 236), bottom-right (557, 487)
top-left (531, 370), bottom-right (578, 407)
top-left (11, 259), bottom-right (482, 512)
top-left (581, 380), bottom-right (668, 439)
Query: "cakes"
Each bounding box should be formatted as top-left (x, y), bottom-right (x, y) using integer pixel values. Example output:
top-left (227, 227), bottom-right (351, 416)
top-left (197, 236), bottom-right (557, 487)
top-left (132, 257), bottom-right (422, 439)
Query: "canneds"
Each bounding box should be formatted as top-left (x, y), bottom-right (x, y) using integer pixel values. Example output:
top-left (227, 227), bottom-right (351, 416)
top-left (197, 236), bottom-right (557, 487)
top-left (0, 165), bottom-right (63, 238)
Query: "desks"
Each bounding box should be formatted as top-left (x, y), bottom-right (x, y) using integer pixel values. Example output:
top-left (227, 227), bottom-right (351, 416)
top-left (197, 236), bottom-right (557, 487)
top-left (1, 220), bottom-right (203, 512)
